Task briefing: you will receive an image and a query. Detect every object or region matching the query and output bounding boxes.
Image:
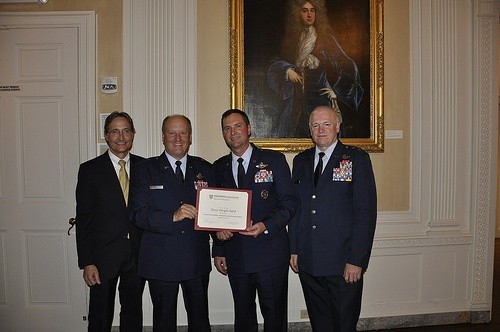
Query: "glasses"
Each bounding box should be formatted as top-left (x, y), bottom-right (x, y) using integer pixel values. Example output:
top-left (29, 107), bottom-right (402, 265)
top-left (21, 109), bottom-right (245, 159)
top-left (107, 127), bottom-right (132, 137)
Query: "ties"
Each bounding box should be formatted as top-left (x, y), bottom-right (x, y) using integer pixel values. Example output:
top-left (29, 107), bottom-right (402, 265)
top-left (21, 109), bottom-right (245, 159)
top-left (314, 152), bottom-right (326, 188)
top-left (175, 161), bottom-right (185, 185)
top-left (236, 157), bottom-right (246, 187)
top-left (118, 160), bottom-right (130, 207)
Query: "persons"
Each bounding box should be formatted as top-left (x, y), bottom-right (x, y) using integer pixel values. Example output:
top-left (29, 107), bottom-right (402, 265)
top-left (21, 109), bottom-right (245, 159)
top-left (209, 108), bottom-right (300, 332)
top-left (75, 111), bottom-right (148, 332)
top-left (258, 0), bottom-right (368, 138)
top-left (288, 105), bottom-right (378, 332)
top-left (128, 114), bottom-right (234, 332)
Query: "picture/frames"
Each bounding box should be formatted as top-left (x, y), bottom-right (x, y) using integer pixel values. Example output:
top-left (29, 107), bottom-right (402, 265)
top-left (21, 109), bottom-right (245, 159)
top-left (230, 0), bottom-right (384, 153)
